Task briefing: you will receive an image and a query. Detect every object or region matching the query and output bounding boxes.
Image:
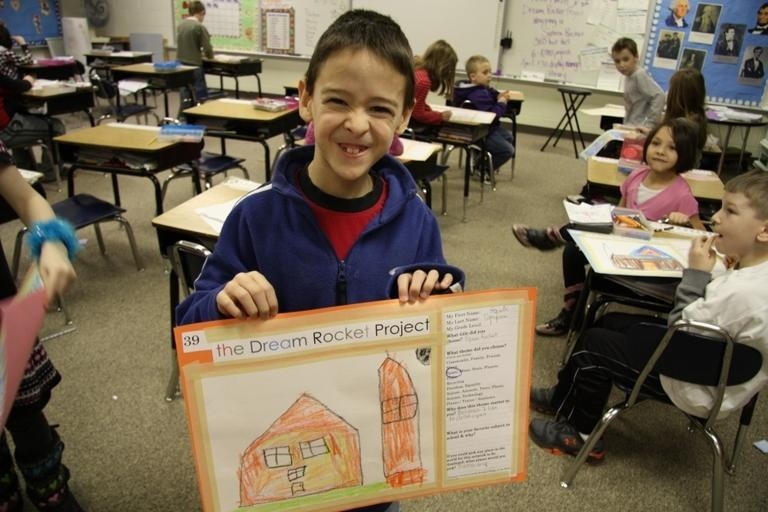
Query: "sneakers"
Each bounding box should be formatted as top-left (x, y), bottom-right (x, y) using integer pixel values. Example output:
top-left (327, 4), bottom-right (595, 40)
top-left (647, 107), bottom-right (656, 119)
top-left (474, 169), bottom-right (491, 185)
top-left (530, 419), bottom-right (603, 465)
top-left (530, 387), bottom-right (557, 416)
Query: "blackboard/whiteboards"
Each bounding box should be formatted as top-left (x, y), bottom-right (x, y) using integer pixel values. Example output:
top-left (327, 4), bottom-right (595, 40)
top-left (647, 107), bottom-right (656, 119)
top-left (0, 0), bottom-right (63, 49)
top-left (492, 0), bottom-right (768, 112)
top-left (171, 0), bottom-right (507, 76)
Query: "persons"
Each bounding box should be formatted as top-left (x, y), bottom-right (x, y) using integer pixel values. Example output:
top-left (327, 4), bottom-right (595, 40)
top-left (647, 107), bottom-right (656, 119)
top-left (512, 117), bottom-right (703, 335)
top-left (530, 169), bottom-right (767, 464)
top-left (3, 147), bottom-right (83, 511)
top-left (0, 26), bottom-right (34, 80)
top-left (652, 0), bottom-right (767, 87)
top-left (172, 12), bottom-right (465, 512)
top-left (448, 55), bottom-right (515, 184)
top-left (611, 37), bottom-right (665, 127)
top-left (0, 73), bottom-right (76, 183)
top-left (400, 38), bottom-right (458, 138)
top-left (567, 69), bottom-right (707, 204)
top-left (176, 1), bottom-right (215, 119)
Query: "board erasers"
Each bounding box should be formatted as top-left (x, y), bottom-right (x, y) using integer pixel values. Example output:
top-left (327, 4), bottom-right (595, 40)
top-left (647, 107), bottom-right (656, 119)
top-left (289, 54), bottom-right (301, 56)
top-left (544, 78), bottom-right (559, 82)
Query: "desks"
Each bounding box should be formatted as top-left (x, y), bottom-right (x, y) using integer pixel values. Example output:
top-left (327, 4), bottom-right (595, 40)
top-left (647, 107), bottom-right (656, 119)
top-left (109, 59), bottom-right (196, 123)
top-left (24, 58), bottom-right (75, 81)
top-left (183, 94), bottom-right (299, 191)
top-left (706, 102), bottom-right (767, 175)
top-left (390, 136), bottom-right (442, 213)
top-left (447, 90), bottom-right (525, 180)
top-left (84, 50), bottom-right (152, 67)
top-left (599, 104), bottom-right (624, 130)
top-left (153, 175), bottom-right (263, 361)
top-left (587, 155), bottom-right (726, 214)
top-left (411, 104), bottom-right (490, 220)
top-left (205, 53), bottom-right (261, 99)
top-left (49, 121), bottom-right (198, 223)
top-left (561, 222), bottom-right (730, 364)
top-left (613, 122), bottom-right (722, 172)
top-left (17, 79), bottom-right (98, 124)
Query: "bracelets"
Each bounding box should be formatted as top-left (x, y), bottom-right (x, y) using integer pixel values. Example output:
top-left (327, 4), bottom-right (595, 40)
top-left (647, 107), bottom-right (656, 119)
top-left (27, 218), bottom-right (77, 260)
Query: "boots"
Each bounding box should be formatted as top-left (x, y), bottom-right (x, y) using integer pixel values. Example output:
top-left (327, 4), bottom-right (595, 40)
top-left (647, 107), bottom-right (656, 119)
top-left (534, 284), bottom-right (585, 335)
top-left (14, 425), bottom-right (82, 511)
top-left (513, 224), bottom-right (566, 250)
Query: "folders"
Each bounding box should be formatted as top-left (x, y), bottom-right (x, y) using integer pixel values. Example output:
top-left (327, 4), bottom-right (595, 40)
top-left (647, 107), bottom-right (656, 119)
top-left (1, 287), bottom-right (47, 436)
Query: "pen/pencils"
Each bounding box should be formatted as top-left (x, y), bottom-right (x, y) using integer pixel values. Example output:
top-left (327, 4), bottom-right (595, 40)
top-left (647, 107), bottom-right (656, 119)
top-left (147, 134), bottom-right (158, 145)
top-left (613, 214), bottom-right (645, 229)
top-left (655, 226), bottom-right (673, 232)
top-left (640, 116), bottom-right (648, 126)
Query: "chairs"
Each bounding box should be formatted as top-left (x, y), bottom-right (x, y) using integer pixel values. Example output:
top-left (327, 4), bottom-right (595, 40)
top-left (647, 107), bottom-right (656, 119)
top-left (162, 116), bottom-right (250, 203)
top-left (75, 60), bottom-right (85, 82)
top-left (443, 98), bottom-right (497, 193)
top-left (166, 240), bottom-right (215, 404)
top-left (557, 318), bottom-right (762, 511)
top-left (177, 61), bottom-right (227, 120)
top-left (399, 131), bottom-right (449, 215)
top-left (9, 184), bottom-right (145, 326)
top-left (270, 126), bottom-right (305, 173)
top-left (93, 79), bottom-right (160, 123)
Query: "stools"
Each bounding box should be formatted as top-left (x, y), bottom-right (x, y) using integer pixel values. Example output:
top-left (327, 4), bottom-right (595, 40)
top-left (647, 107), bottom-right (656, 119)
top-left (538, 87), bottom-right (592, 160)
